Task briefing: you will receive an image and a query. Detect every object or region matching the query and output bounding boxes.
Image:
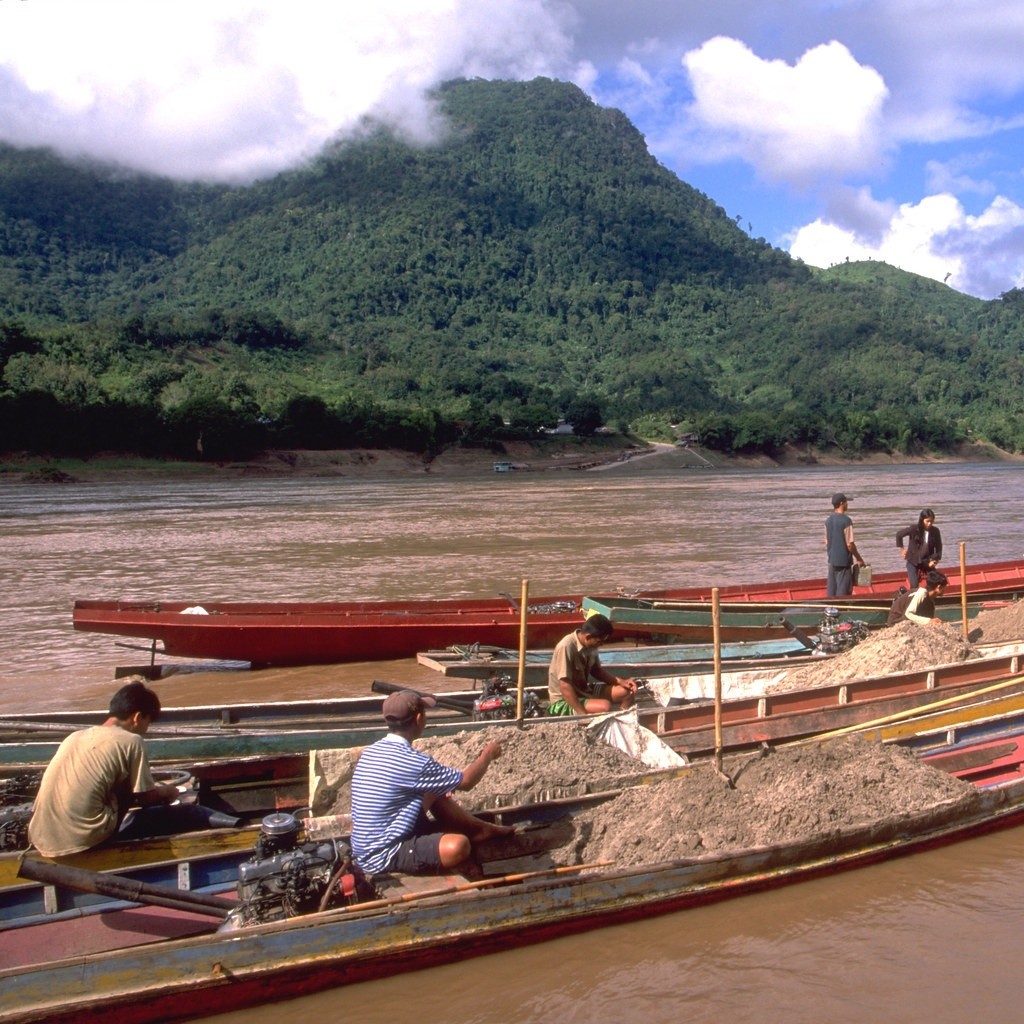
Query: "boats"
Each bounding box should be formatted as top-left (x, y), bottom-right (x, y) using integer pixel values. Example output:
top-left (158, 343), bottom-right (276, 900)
top-left (0, 556), bottom-right (1023, 1024)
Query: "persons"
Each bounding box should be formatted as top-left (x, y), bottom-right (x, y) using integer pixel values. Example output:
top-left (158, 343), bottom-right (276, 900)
top-left (350, 691), bottom-right (516, 882)
top-left (888, 571), bottom-right (949, 627)
top-left (27, 683), bottom-right (180, 859)
top-left (548, 613), bottom-right (638, 716)
top-left (824, 493), bottom-right (866, 596)
top-left (896, 509), bottom-right (942, 589)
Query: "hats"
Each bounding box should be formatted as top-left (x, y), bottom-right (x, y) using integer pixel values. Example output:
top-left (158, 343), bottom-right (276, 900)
top-left (383, 689), bottom-right (437, 724)
top-left (832, 492), bottom-right (853, 504)
